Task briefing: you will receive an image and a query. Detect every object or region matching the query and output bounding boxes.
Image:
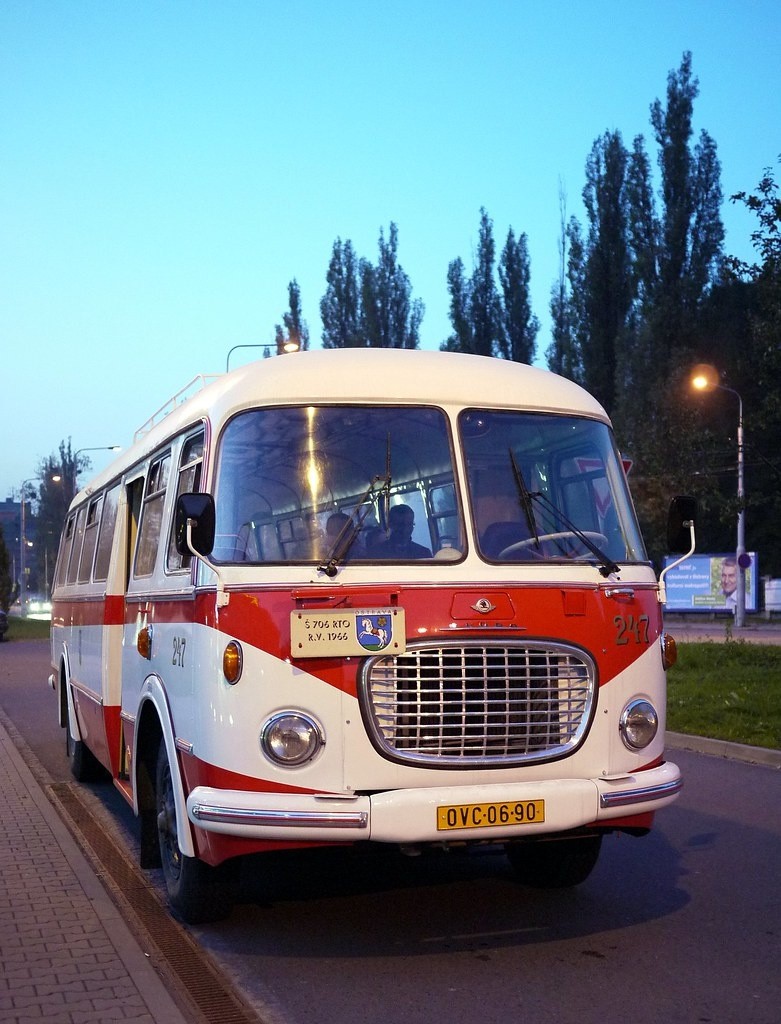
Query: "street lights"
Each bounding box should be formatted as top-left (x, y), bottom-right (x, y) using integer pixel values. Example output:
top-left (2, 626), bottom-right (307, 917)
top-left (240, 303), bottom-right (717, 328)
top-left (224, 339), bottom-right (299, 374)
top-left (13, 538), bottom-right (34, 592)
top-left (18, 472), bottom-right (62, 617)
top-left (692, 375), bottom-right (745, 629)
top-left (72, 444), bottom-right (123, 495)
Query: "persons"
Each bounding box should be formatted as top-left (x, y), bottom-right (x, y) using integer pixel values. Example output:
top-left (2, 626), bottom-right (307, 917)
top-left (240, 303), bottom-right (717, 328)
top-left (714, 558), bottom-right (751, 609)
top-left (291, 504), bottom-right (433, 560)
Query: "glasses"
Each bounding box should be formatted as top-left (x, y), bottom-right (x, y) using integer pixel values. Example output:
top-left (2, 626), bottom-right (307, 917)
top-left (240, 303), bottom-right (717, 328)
top-left (394, 522), bottom-right (416, 528)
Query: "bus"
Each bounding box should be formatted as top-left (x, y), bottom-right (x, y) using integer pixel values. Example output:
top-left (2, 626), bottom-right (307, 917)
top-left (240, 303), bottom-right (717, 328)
top-left (45, 344), bottom-right (697, 916)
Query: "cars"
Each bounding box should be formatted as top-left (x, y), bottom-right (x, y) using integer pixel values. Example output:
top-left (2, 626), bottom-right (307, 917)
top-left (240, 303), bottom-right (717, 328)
top-left (8, 593), bottom-right (51, 615)
